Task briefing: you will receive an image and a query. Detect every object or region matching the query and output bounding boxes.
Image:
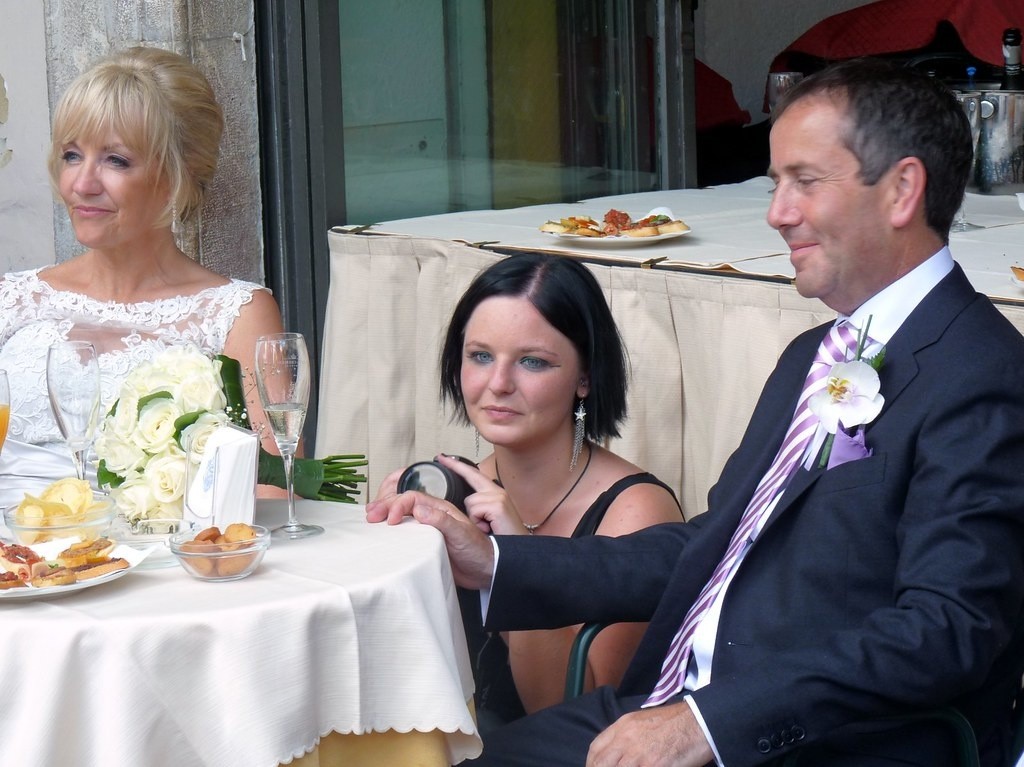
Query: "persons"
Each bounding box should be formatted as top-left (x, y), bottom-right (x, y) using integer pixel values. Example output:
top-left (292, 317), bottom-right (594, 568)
top-left (0, 44), bottom-right (292, 501)
top-left (376, 247), bottom-right (697, 735)
top-left (364, 54), bottom-right (1024, 767)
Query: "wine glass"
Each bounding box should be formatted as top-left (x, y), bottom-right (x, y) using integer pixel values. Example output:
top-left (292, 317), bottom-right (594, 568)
top-left (47, 340), bottom-right (102, 480)
top-left (254, 332), bottom-right (325, 541)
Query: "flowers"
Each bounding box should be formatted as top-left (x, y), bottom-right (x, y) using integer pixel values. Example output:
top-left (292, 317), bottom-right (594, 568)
top-left (92, 344), bottom-right (369, 535)
top-left (807, 361), bottom-right (886, 433)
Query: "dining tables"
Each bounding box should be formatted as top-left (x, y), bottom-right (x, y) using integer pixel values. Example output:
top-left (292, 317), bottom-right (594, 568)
top-left (0, 500), bottom-right (485, 767)
top-left (312, 174), bottom-right (1024, 523)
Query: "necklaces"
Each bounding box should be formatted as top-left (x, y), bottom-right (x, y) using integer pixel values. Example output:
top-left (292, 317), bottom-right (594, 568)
top-left (495, 438), bottom-right (592, 535)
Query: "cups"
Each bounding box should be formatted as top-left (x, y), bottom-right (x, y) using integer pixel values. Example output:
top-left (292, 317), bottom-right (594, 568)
top-left (0, 370), bottom-right (11, 455)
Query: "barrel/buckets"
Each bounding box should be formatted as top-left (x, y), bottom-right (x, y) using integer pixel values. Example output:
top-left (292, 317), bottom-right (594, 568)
top-left (945, 84), bottom-right (1024, 196)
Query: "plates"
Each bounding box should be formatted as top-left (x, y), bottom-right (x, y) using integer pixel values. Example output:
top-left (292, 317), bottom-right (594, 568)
top-left (541, 227), bottom-right (692, 247)
top-left (0, 543), bottom-right (133, 602)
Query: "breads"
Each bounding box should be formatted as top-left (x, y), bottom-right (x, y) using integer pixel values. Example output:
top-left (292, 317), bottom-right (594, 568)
top-left (177, 522), bottom-right (258, 576)
top-left (540, 212), bottom-right (691, 237)
top-left (0, 538), bottom-right (129, 591)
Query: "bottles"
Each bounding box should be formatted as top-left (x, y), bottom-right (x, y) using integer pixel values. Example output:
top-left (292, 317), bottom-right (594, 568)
top-left (998, 28), bottom-right (1024, 91)
top-left (963, 66), bottom-right (985, 90)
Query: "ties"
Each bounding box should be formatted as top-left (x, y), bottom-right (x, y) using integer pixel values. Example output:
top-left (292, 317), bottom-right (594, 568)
top-left (638, 320), bottom-right (872, 710)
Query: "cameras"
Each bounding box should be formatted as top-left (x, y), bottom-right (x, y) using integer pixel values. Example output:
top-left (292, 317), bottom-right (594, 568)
top-left (396, 452), bottom-right (505, 514)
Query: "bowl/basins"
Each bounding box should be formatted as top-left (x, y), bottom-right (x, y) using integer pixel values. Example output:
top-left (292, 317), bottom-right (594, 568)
top-left (168, 524), bottom-right (271, 582)
top-left (4, 496), bottom-right (116, 547)
top-left (100, 519), bottom-right (200, 572)
